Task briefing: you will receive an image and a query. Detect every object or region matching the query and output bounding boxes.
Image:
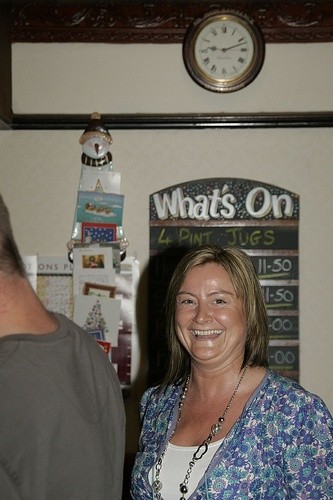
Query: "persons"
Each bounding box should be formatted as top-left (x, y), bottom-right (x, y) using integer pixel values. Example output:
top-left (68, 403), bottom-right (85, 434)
top-left (0, 195), bottom-right (124, 500)
top-left (130, 245), bottom-right (333, 500)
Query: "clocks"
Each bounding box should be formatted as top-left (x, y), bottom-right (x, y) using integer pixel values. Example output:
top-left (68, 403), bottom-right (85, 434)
top-left (182, 8), bottom-right (267, 94)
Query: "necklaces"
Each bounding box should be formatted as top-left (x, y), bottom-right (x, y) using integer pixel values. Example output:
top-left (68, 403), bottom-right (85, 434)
top-left (153, 361), bottom-right (249, 500)
